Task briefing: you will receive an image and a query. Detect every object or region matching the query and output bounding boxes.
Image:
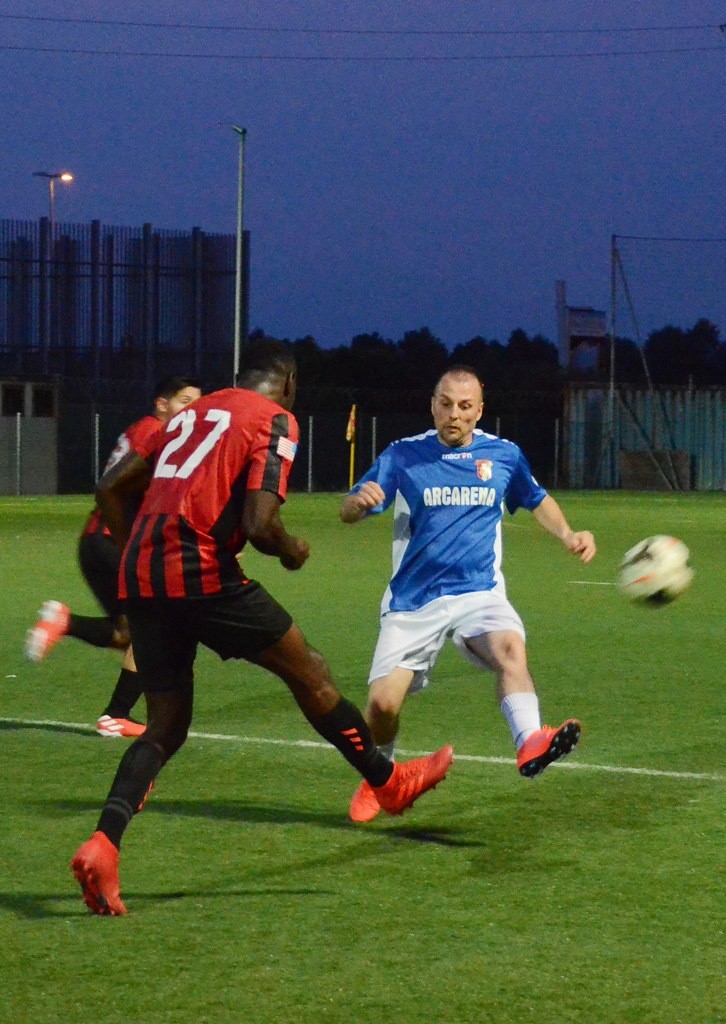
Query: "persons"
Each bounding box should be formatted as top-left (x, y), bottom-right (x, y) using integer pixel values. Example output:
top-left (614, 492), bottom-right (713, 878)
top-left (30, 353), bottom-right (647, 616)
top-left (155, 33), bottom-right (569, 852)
top-left (340, 366), bottom-right (596, 821)
top-left (69, 344), bottom-right (455, 914)
top-left (26, 377), bottom-right (202, 739)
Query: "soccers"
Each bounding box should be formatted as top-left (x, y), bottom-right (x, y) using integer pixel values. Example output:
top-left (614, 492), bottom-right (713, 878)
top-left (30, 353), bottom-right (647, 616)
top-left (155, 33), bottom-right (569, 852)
top-left (620, 534), bottom-right (698, 609)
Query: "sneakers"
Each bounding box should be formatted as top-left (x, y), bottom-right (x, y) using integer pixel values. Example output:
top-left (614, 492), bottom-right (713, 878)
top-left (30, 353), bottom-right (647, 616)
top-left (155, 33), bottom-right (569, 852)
top-left (517, 719), bottom-right (581, 778)
top-left (134, 780), bottom-right (154, 812)
top-left (96, 713), bottom-right (147, 738)
top-left (25, 600), bottom-right (70, 664)
top-left (371, 744), bottom-right (454, 815)
top-left (350, 779), bottom-right (381, 822)
top-left (72, 830), bottom-right (128, 917)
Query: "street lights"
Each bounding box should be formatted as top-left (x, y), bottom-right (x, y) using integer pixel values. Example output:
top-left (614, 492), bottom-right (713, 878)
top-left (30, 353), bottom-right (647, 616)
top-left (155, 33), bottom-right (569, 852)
top-left (31, 171), bottom-right (74, 255)
top-left (232, 125), bottom-right (248, 389)
